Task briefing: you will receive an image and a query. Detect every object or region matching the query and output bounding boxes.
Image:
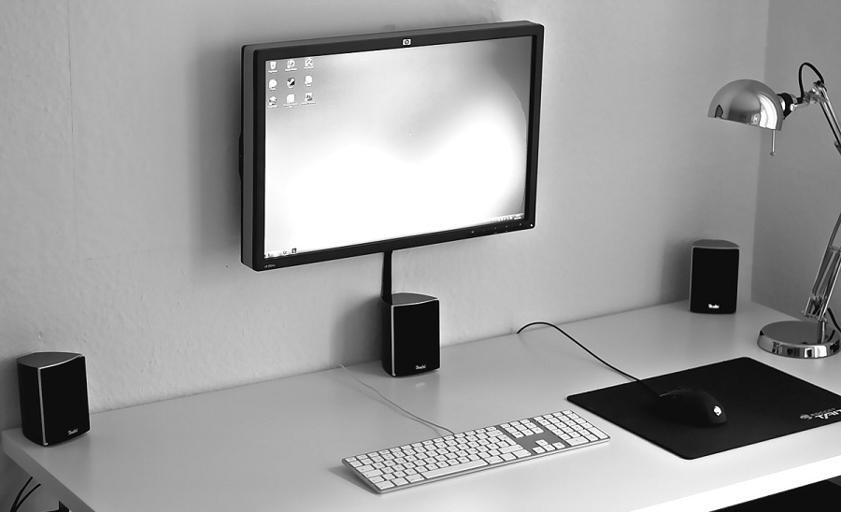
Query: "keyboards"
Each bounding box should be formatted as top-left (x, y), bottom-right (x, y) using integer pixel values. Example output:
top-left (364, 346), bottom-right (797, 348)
top-left (342, 410), bottom-right (611, 494)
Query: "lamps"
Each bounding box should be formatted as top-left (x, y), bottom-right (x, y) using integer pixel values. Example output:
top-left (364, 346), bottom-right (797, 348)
top-left (711, 61), bottom-right (840, 360)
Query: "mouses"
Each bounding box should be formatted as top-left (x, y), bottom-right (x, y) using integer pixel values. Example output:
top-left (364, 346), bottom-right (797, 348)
top-left (658, 388), bottom-right (727, 426)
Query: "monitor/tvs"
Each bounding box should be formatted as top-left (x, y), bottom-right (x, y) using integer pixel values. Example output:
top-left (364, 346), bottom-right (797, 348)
top-left (239, 20), bottom-right (546, 272)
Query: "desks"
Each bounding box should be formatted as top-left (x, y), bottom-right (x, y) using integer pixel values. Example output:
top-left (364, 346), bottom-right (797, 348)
top-left (0, 300), bottom-right (841, 512)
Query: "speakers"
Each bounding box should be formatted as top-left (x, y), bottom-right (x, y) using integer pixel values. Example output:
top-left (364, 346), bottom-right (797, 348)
top-left (15, 351), bottom-right (90, 446)
top-left (382, 293), bottom-right (441, 378)
top-left (688, 239), bottom-right (740, 315)
